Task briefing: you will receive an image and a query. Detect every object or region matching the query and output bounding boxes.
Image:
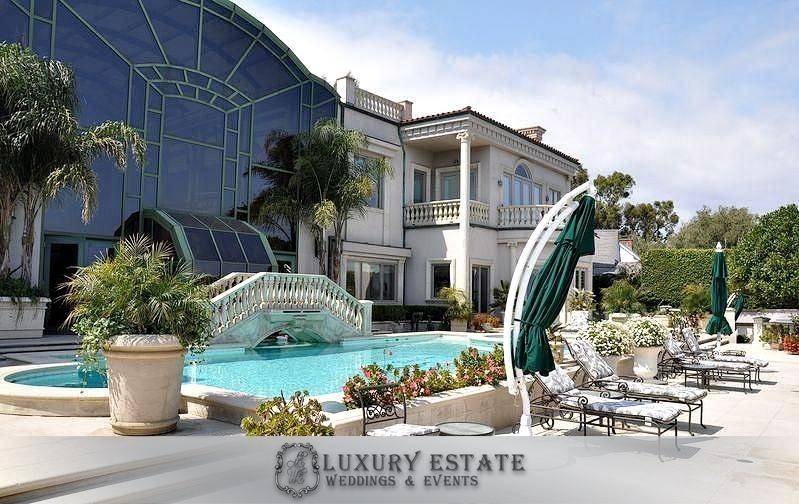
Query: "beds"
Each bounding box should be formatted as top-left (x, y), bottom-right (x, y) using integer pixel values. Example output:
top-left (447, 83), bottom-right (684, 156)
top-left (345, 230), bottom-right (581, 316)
top-left (435, 421), bottom-right (496, 436)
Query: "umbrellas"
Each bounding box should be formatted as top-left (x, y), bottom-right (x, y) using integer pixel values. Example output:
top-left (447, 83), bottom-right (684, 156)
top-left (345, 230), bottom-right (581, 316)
top-left (727, 290), bottom-right (745, 319)
top-left (704, 242), bottom-right (733, 356)
top-left (503, 179), bottom-right (598, 435)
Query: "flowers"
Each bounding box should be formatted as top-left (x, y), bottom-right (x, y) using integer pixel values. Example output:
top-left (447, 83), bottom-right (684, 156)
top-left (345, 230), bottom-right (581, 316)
top-left (341, 343), bottom-right (507, 410)
top-left (475, 312), bottom-right (499, 327)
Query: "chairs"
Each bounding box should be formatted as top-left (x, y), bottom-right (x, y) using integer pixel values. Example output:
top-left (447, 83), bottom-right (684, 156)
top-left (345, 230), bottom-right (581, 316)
top-left (354, 382), bottom-right (441, 437)
top-left (511, 328), bottom-right (770, 464)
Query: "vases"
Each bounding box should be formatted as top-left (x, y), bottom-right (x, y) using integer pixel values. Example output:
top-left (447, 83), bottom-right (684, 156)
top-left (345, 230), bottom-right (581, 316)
top-left (480, 322), bottom-right (492, 332)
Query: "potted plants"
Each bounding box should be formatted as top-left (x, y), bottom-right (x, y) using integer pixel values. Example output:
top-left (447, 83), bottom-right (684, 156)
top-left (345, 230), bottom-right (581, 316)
top-left (55, 230), bottom-right (223, 437)
top-left (758, 323), bottom-right (790, 350)
top-left (566, 287), bottom-right (598, 325)
top-left (0, 263), bottom-right (52, 340)
top-left (437, 281), bottom-right (474, 331)
